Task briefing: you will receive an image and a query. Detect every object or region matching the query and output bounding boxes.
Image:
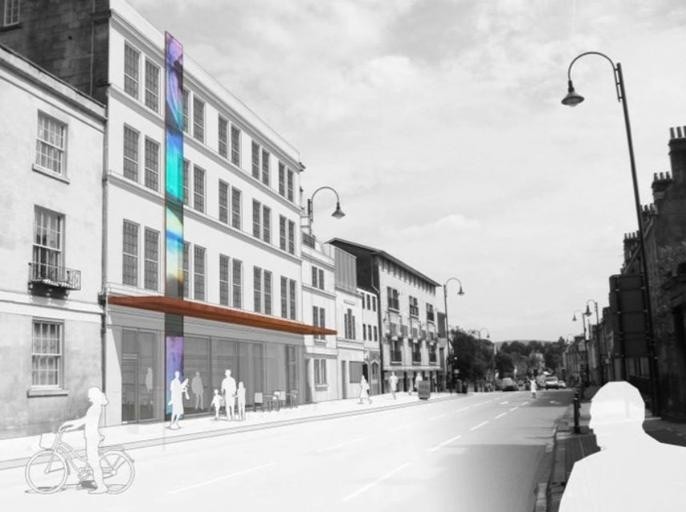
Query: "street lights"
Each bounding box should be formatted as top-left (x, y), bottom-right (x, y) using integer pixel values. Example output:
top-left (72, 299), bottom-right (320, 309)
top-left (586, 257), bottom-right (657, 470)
top-left (561, 50), bottom-right (661, 421)
top-left (584, 298), bottom-right (606, 387)
top-left (442, 275), bottom-right (465, 393)
top-left (571, 309), bottom-right (588, 386)
top-left (477, 327), bottom-right (490, 349)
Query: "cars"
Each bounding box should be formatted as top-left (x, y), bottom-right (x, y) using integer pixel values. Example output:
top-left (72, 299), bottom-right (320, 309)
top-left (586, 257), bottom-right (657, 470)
top-left (499, 373), bottom-right (568, 390)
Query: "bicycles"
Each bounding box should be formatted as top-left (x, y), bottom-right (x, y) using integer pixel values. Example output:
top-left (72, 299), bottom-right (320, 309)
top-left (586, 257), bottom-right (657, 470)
top-left (23, 426), bottom-right (135, 496)
